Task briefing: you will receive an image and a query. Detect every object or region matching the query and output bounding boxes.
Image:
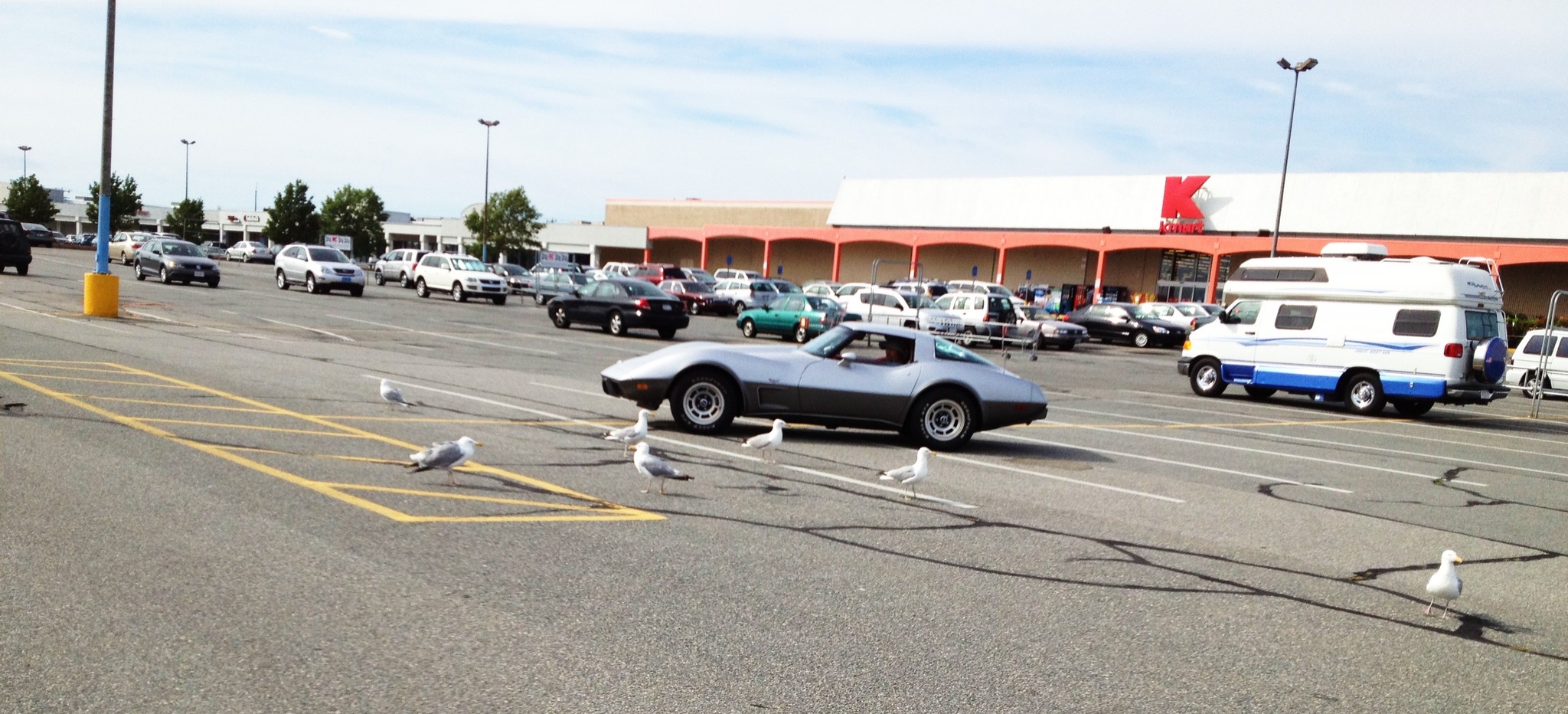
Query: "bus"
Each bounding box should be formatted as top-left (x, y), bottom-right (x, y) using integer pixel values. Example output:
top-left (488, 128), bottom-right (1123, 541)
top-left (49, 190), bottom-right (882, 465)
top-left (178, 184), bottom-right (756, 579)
top-left (274, 240), bottom-right (366, 298)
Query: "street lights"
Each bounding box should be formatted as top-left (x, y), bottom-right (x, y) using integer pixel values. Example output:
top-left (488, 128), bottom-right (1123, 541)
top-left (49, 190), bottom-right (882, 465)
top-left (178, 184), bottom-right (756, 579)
top-left (181, 139), bottom-right (196, 200)
top-left (1269, 57), bottom-right (1318, 258)
top-left (19, 145), bottom-right (32, 186)
top-left (478, 119), bottom-right (499, 262)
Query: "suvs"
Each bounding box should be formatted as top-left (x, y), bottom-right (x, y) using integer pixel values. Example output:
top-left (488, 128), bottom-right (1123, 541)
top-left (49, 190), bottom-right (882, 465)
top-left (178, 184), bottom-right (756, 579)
top-left (374, 248), bottom-right (433, 289)
top-left (414, 252), bottom-right (508, 305)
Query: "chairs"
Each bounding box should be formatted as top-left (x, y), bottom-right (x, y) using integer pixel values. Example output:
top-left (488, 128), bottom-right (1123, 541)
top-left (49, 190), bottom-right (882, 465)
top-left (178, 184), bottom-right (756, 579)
top-left (171, 247), bottom-right (190, 255)
top-left (818, 304), bottom-right (828, 309)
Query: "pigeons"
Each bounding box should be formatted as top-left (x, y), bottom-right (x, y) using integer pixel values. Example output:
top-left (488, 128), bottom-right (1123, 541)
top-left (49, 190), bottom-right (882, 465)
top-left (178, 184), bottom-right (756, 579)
top-left (1423, 550), bottom-right (1464, 619)
top-left (875, 447), bottom-right (938, 500)
top-left (740, 418), bottom-right (792, 464)
top-left (409, 435), bottom-right (483, 485)
top-left (600, 409), bottom-right (695, 496)
top-left (379, 378), bottom-right (417, 411)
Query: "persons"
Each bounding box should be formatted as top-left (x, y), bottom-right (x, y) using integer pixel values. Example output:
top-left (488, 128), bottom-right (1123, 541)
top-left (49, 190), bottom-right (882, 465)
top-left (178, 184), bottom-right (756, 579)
top-left (879, 338), bottom-right (904, 366)
top-left (1045, 290), bottom-right (1052, 304)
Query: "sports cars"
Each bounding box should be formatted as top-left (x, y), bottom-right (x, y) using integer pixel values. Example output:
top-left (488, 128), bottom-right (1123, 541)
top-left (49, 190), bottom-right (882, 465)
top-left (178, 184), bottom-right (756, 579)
top-left (600, 317), bottom-right (1050, 452)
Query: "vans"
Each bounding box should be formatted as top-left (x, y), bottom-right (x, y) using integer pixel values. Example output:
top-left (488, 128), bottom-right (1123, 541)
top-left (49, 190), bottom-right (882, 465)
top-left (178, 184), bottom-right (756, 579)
top-left (1177, 242), bottom-right (1512, 418)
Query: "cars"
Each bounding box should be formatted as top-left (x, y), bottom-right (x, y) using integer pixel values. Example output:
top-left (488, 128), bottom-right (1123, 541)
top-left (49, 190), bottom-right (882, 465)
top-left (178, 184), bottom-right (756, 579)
top-left (1505, 327), bottom-right (1568, 401)
top-left (66, 231), bottom-right (183, 253)
top-left (546, 276), bottom-right (690, 340)
top-left (134, 238), bottom-right (220, 289)
top-left (224, 240), bottom-right (273, 264)
top-left (20, 222), bottom-right (55, 249)
top-left (0, 212), bottom-right (33, 276)
top-left (196, 240), bottom-right (229, 260)
top-left (50, 230), bottom-right (70, 245)
top-left (108, 232), bottom-right (158, 266)
top-left (737, 292), bottom-right (863, 344)
top-left (530, 259), bottom-right (1229, 353)
top-left (485, 262), bottom-right (538, 296)
